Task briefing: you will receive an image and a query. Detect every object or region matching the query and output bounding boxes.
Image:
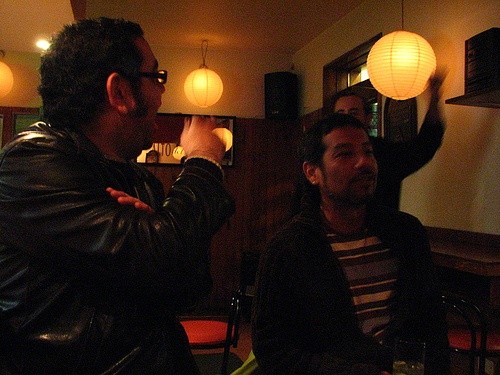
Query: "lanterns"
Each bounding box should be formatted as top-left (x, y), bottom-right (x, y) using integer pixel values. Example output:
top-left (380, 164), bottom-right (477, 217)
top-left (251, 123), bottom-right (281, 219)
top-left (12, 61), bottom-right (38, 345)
top-left (0, 61), bottom-right (14, 98)
top-left (183, 65), bottom-right (225, 108)
top-left (368, 31), bottom-right (434, 100)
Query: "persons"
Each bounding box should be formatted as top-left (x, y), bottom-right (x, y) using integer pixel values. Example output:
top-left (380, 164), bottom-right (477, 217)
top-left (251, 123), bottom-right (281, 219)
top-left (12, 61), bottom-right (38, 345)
top-left (251, 113), bottom-right (452, 375)
top-left (0, 18), bottom-right (235, 375)
top-left (289, 66), bottom-right (451, 220)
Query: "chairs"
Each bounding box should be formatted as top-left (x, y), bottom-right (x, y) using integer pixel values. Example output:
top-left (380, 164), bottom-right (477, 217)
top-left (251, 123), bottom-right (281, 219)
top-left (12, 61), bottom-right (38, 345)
top-left (429, 294), bottom-right (500, 375)
top-left (180, 281), bottom-right (247, 375)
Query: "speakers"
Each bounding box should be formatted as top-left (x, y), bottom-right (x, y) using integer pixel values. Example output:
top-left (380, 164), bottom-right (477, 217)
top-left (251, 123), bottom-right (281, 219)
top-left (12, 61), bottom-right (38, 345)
top-left (263, 71), bottom-right (298, 120)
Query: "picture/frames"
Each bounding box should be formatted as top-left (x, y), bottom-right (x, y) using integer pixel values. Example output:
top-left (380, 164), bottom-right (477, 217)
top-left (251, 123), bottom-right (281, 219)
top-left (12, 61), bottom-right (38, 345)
top-left (12, 112), bottom-right (40, 141)
top-left (381, 97), bottom-right (418, 143)
top-left (0, 114), bottom-right (4, 149)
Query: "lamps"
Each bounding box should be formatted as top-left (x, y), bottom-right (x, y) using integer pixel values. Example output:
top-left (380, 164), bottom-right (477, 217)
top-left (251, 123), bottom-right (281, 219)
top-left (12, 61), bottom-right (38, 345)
top-left (366, 0), bottom-right (437, 101)
top-left (183, 39), bottom-right (224, 109)
top-left (0, 50), bottom-right (14, 98)
top-left (212, 128), bottom-right (233, 151)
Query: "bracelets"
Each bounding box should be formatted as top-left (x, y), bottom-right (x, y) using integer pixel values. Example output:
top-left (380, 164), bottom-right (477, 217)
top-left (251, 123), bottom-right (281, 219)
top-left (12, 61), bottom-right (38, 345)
top-left (184, 156), bottom-right (224, 174)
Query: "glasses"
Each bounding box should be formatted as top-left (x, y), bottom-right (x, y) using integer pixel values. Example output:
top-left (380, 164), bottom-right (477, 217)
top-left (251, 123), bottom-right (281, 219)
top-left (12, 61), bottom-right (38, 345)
top-left (119, 69), bottom-right (167, 84)
